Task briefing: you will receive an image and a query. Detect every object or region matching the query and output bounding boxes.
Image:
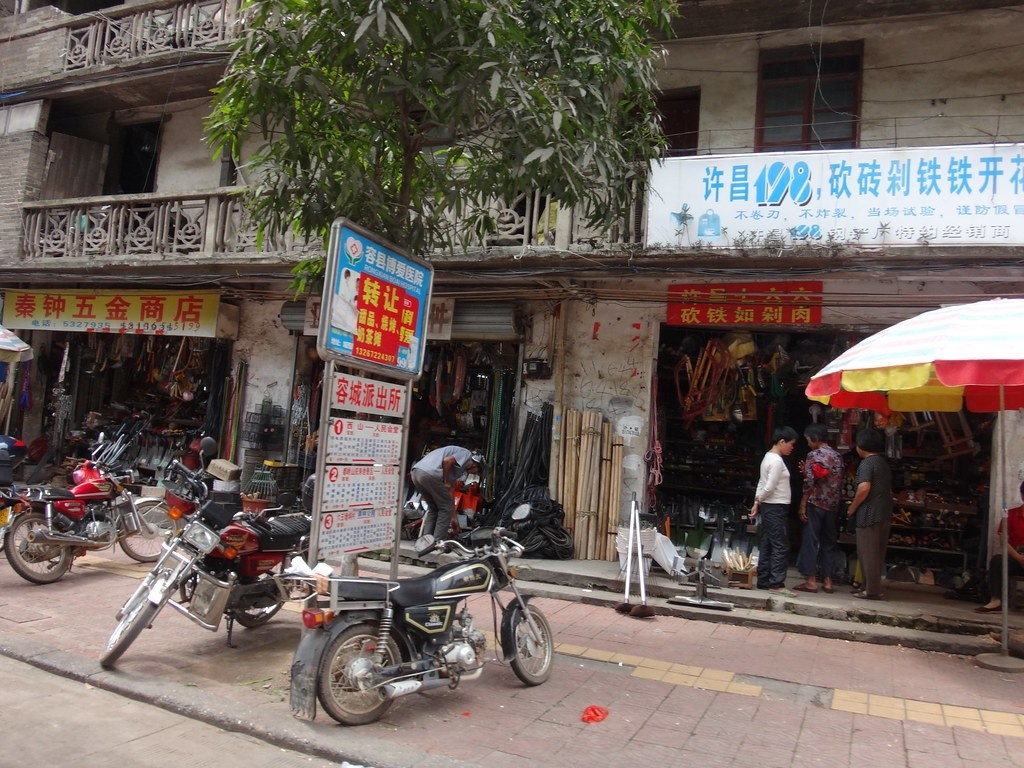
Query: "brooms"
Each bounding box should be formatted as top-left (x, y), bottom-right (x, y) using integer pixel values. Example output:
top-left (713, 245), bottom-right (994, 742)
top-left (615, 490), bottom-right (656, 618)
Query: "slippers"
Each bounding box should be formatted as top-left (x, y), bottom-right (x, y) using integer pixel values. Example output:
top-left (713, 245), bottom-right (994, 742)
top-left (792, 584), bottom-right (818, 593)
top-left (769, 587), bottom-right (797, 596)
top-left (822, 585), bottom-right (833, 593)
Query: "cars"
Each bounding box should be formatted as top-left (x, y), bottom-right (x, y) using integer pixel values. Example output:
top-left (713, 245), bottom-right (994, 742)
top-left (0, 435), bottom-right (26, 485)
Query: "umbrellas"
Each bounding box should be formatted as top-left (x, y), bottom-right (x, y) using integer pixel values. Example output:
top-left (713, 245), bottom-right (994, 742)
top-left (0, 320), bottom-right (34, 363)
top-left (805, 298), bottom-right (1024, 675)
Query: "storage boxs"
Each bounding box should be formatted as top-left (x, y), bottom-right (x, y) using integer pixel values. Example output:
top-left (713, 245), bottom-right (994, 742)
top-left (206, 459), bottom-right (243, 482)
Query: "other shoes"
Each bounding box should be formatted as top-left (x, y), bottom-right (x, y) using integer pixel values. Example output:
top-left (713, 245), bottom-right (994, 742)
top-left (974, 605), bottom-right (1002, 613)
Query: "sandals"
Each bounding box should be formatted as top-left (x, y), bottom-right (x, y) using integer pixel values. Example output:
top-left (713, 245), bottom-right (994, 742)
top-left (850, 589), bottom-right (862, 593)
top-left (853, 591), bottom-right (885, 599)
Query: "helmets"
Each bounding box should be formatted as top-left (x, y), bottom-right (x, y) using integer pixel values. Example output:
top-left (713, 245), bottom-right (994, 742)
top-left (472, 452), bottom-right (485, 472)
top-left (165, 489), bottom-right (200, 515)
top-left (72, 469), bottom-right (100, 484)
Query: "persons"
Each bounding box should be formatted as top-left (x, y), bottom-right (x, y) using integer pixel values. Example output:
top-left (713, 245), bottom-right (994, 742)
top-left (847, 428), bottom-right (896, 601)
top-left (974, 483), bottom-right (1024, 613)
top-left (411, 445), bottom-right (487, 540)
top-left (746, 427), bottom-right (798, 598)
top-left (792, 424), bottom-right (845, 596)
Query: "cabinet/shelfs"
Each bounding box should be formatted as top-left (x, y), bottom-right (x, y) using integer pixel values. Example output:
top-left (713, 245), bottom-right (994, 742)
top-left (888, 499), bottom-right (978, 551)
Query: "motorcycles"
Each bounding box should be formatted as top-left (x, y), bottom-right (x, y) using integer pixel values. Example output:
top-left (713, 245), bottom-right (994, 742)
top-left (0, 430), bottom-right (183, 584)
top-left (98, 434), bottom-right (314, 667)
top-left (269, 500), bottom-right (554, 727)
